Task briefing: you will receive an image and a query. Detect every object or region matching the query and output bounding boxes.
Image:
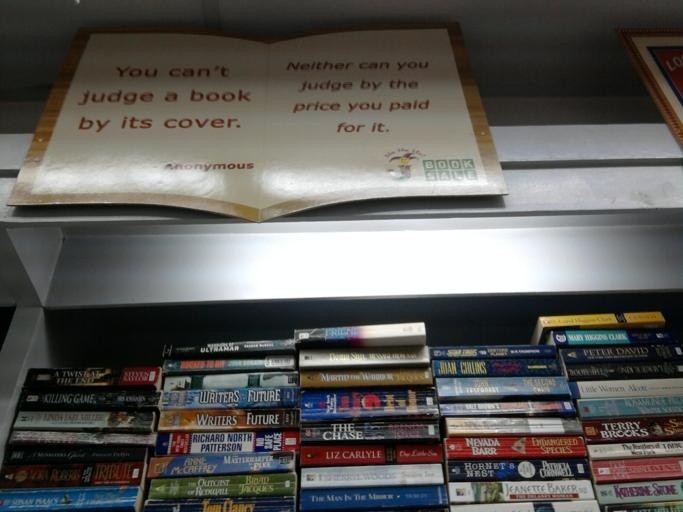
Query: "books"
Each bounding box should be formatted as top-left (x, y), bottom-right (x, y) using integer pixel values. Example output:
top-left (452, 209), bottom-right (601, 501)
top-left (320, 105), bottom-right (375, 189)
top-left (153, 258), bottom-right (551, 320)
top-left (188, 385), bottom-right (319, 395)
top-left (0, 307), bottom-right (683, 510)
